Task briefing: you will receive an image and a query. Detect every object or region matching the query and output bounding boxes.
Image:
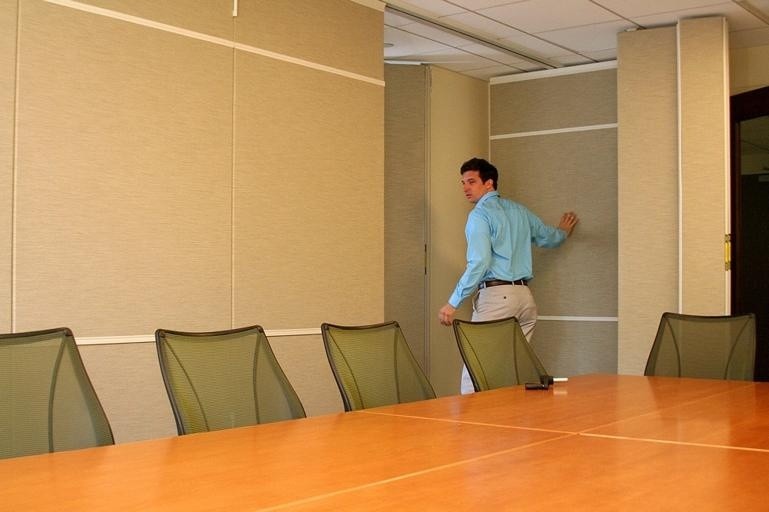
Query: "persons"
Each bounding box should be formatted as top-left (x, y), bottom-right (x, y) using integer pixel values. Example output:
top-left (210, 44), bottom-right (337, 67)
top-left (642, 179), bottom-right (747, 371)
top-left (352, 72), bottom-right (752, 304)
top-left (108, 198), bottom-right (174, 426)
top-left (438, 158), bottom-right (579, 395)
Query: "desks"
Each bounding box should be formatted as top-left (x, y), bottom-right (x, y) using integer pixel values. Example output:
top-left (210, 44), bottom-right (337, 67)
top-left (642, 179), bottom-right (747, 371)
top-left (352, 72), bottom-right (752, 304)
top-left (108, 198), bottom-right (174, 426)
top-left (1, 373), bottom-right (768, 512)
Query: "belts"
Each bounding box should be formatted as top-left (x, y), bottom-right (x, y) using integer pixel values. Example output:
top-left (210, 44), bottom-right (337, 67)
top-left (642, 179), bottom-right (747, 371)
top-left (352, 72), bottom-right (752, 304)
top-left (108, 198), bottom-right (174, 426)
top-left (479, 279), bottom-right (528, 290)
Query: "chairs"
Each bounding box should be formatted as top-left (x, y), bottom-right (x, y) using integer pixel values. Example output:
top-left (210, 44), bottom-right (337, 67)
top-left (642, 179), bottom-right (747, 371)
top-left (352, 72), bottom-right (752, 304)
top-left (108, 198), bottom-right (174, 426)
top-left (154, 323), bottom-right (307, 435)
top-left (454, 319), bottom-right (555, 392)
top-left (643, 313), bottom-right (756, 380)
top-left (318, 321), bottom-right (437, 414)
top-left (0, 326), bottom-right (115, 458)
